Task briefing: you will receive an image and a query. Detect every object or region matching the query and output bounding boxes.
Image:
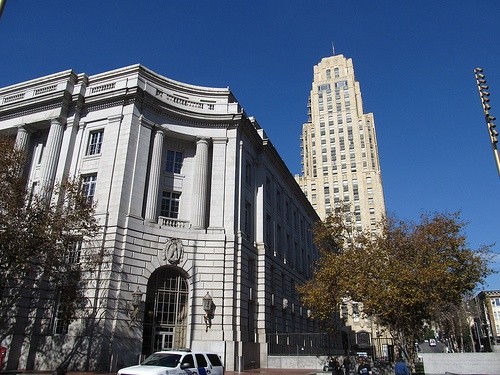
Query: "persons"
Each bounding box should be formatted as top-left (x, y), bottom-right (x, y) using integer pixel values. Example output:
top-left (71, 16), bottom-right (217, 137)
top-left (0, 343), bottom-right (9, 370)
top-left (358, 357), bottom-right (372, 375)
top-left (395, 357), bottom-right (410, 375)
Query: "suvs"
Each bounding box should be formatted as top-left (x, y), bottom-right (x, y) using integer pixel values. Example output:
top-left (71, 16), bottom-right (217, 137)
top-left (115, 349), bottom-right (225, 375)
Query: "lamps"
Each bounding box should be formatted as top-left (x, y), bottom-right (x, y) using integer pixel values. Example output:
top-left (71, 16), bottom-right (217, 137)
top-left (127, 285), bottom-right (143, 326)
top-left (203, 290), bottom-right (214, 332)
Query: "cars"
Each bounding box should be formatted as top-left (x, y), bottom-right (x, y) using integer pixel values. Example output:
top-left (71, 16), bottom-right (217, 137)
top-left (429, 338), bottom-right (436, 347)
top-left (442, 348), bottom-right (455, 354)
